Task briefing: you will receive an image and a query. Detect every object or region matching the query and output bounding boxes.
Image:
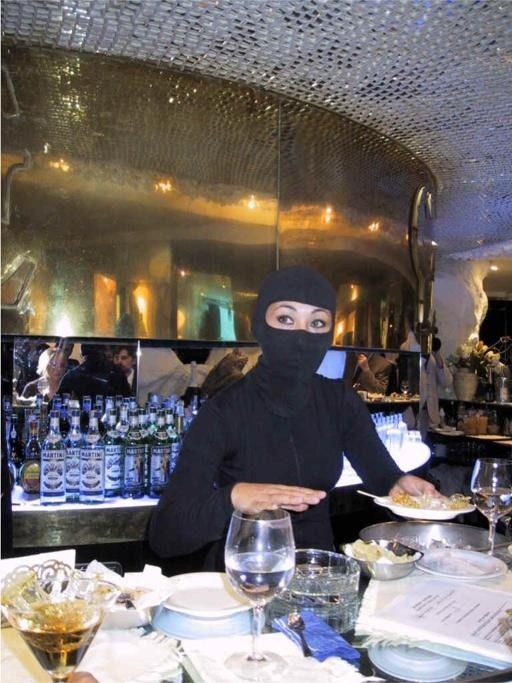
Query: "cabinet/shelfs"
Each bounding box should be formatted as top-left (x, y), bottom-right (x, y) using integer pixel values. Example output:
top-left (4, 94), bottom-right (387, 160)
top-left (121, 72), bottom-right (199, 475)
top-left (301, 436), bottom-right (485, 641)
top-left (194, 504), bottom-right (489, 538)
top-left (1, 332), bottom-right (416, 555)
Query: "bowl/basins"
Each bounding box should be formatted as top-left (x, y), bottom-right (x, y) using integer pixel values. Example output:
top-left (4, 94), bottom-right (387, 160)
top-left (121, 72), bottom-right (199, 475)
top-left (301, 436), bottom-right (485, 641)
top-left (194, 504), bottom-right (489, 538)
top-left (360, 520), bottom-right (511, 560)
top-left (343, 538), bottom-right (421, 582)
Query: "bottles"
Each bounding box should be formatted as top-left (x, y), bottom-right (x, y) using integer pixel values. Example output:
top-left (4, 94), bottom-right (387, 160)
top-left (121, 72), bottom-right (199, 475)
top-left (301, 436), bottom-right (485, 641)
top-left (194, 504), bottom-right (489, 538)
top-left (446, 401), bottom-right (500, 432)
top-left (7, 392), bottom-right (208, 506)
top-left (485, 366), bottom-right (497, 401)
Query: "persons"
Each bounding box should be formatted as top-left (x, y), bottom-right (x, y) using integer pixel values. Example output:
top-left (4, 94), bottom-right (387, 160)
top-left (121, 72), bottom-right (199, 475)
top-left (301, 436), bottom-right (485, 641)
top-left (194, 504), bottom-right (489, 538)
top-left (14, 338), bottom-right (248, 406)
top-left (146, 266), bottom-right (447, 577)
top-left (353, 348), bottom-right (397, 395)
top-left (403, 319), bottom-right (454, 443)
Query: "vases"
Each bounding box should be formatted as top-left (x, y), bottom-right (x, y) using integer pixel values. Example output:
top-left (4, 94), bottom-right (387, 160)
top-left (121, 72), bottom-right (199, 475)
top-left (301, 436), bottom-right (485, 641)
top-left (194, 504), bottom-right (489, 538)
top-left (452, 367), bottom-right (477, 402)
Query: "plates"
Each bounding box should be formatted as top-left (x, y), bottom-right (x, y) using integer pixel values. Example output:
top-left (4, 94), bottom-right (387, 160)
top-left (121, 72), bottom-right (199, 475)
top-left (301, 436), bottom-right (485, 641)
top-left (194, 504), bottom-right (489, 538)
top-left (374, 495), bottom-right (476, 520)
top-left (159, 570), bottom-right (261, 621)
top-left (90, 569), bottom-right (176, 610)
top-left (415, 549), bottom-right (508, 581)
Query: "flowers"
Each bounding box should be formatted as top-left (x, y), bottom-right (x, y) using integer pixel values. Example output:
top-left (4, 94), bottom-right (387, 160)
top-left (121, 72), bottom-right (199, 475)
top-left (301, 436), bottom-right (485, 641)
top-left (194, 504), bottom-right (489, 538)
top-left (444, 336), bottom-right (512, 375)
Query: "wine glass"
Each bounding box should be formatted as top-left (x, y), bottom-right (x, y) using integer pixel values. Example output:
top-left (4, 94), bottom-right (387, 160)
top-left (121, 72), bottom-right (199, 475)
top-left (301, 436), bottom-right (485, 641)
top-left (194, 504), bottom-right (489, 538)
top-left (224, 505), bottom-right (296, 679)
top-left (400, 379), bottom-right (411, 399)
top-left (470, 459), bottom-right (512, 564)
top-left (37, 375), bottom-right (51, 398)
top-left (4, 579), bottom-right (109, 682)
